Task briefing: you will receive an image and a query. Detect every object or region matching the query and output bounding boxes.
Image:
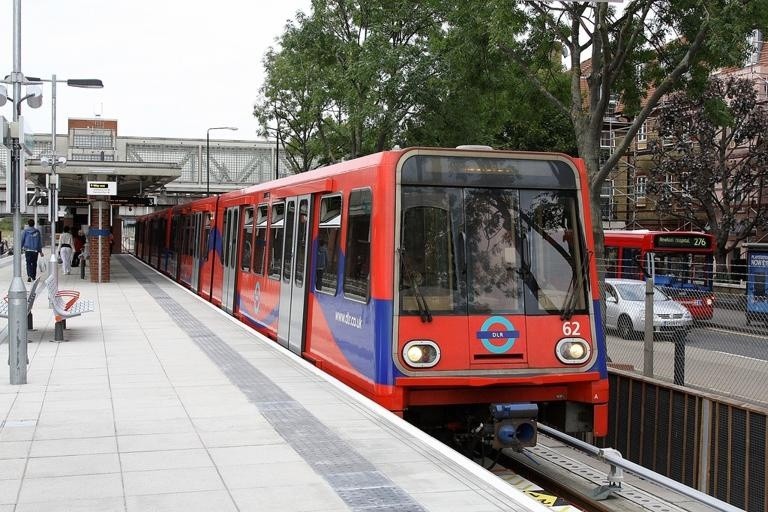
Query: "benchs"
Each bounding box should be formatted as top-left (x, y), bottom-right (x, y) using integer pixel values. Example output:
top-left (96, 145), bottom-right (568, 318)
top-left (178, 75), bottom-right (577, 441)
top-left (45, 275), bottom-right (94, 342)
top-left (0, 275), bottom-right (41, 329)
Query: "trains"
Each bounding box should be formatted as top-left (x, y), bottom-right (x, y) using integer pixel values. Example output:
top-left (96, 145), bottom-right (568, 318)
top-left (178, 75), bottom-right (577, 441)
top-left (133, 141), bottom-right (610, 450)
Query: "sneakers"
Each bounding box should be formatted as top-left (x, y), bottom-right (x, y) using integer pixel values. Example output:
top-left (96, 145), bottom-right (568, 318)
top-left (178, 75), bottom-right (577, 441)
top-left (28, 277), bottom-right (36, 282)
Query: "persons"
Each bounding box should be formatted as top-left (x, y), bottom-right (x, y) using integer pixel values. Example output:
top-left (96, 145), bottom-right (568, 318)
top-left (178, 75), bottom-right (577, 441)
top-left (55, 223), bottom-right (114, 275)
top-left (20, 218), bottom-right (45, 283)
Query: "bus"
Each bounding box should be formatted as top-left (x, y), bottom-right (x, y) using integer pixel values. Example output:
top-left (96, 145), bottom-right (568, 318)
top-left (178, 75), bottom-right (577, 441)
top-left (604, 229), bottom-right (716, 321)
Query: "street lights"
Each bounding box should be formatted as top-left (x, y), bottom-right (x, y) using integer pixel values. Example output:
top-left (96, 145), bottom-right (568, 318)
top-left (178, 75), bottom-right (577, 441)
top-left (0, 0), bottom-right (106, 385)
top-left (205, 126), bottom-right (238, 198)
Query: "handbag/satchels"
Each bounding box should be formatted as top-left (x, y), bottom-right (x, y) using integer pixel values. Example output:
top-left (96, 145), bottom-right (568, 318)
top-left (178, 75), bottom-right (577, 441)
top-left (56, 252), bottom-right (63, 264)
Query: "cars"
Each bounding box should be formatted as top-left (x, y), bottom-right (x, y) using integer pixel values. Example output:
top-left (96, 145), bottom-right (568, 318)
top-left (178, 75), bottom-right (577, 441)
top-left (605, 277), bottom-right (693, 341)
